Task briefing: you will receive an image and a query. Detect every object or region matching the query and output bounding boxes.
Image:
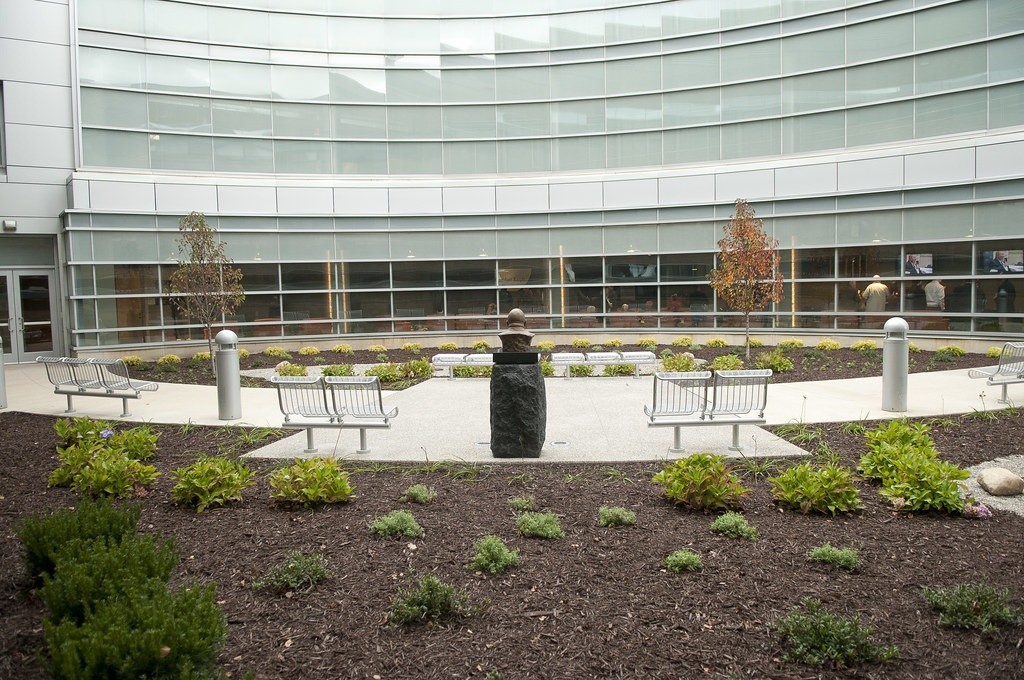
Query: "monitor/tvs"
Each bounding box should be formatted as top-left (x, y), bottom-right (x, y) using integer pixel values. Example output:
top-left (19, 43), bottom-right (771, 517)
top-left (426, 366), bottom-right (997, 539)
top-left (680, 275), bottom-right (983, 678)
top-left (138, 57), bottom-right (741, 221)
top-left (979, 249), bottom-right (1024, 274)
top-left (896, 253), bottom-right (935, 277)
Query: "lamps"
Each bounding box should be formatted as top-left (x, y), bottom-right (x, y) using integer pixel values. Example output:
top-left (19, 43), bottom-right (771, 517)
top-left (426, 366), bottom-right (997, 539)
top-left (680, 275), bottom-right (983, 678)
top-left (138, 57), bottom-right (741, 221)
top-left (3, 220), bottom-right (17, 231)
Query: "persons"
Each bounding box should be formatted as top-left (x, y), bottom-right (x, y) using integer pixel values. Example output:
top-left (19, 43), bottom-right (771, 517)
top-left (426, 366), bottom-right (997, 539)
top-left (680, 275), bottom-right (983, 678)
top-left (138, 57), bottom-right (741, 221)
top-left (486, 303), bottom-right (496, 314)
top-left (672, 286), bottom-right (708, 327)
top-left (948, 280), bottom-right (1016, 331)
top-left (498, 308), bottom-right (534, 352)
top-left (909, 279), bottom-right (946, 311)
top-left (905, 254), bottom-right (923, 275)
top-left (987, 250), bottom-right (1010, 273)
top-left (607, 287), bottom-right (617, 312)
top-left (838, 274), bottom-right (900, 311)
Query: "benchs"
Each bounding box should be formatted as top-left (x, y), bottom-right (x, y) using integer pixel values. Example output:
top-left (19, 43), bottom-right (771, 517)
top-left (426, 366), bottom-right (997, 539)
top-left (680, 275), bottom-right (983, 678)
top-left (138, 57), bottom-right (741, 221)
top-left (427, 312), bottom-right (757, 330)
top-left (36, 356), bottom-right (158, 417)
top-left (820, 310), bottom-right (947, 330)
top-left (253, 318), bottom-right (283, 336)
top-left (432, 353), bottom-right (541, 381)
top-left (271, 376), bottom-right (399, 454)
top-left (968, 342), bottom-right (1024, 403)
top-left (300, 317), bottom-right (332, 334)
top-left (205, 319), bottom-right (238, 340)
top-left (643, 368), bottom-right (773, 452)
top-left (549, 351), bottom-right (655, 380)
top-left (380, 322), bottom-right (411, 332)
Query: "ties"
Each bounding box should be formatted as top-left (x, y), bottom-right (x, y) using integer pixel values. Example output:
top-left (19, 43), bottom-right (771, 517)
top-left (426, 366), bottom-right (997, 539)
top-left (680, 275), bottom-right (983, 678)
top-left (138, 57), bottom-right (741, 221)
top-left (1001, 261), bottom-right (1003, 266)
top-left (912, 263), bottom-right (915, 268)
